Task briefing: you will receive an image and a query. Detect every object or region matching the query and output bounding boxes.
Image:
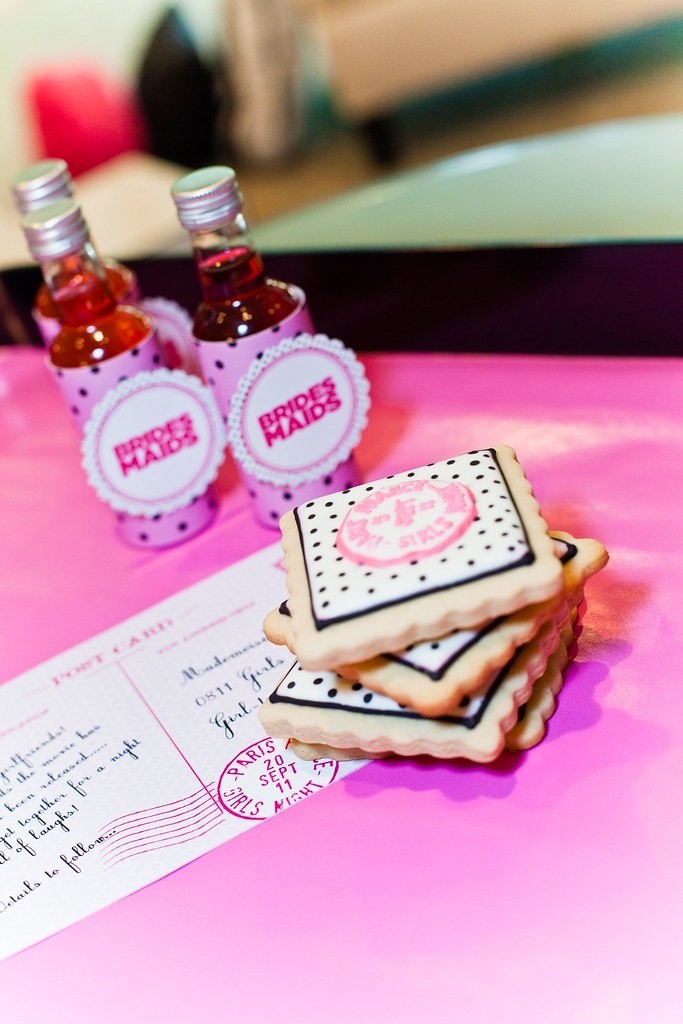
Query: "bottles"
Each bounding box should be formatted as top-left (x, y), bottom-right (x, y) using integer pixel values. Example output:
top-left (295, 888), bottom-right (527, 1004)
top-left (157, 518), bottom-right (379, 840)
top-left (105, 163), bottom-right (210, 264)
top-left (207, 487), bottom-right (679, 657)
top-left (18, 199), bottom-right (220, 546)
top-left (171, 165), bottom-right (363, 531)
top-left (12, 159), bottom-right (140, 350)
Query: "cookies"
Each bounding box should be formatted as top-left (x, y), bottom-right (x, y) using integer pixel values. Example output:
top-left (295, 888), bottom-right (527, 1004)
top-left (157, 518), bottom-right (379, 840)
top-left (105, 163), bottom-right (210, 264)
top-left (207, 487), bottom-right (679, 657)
top-left (260, 447), bottom-right (611, 762)
